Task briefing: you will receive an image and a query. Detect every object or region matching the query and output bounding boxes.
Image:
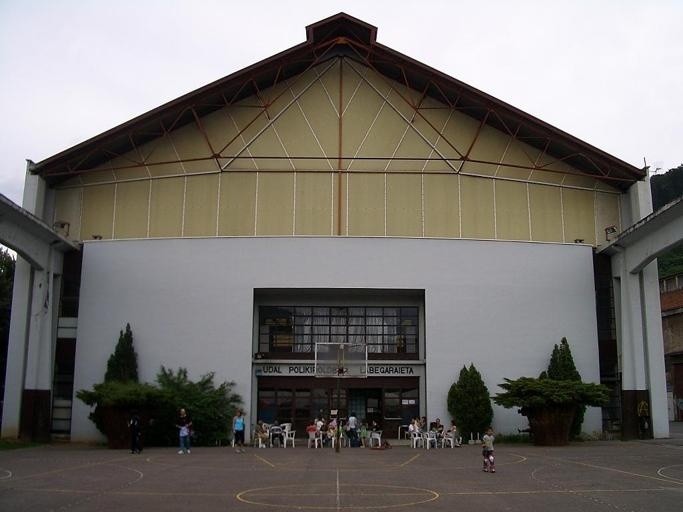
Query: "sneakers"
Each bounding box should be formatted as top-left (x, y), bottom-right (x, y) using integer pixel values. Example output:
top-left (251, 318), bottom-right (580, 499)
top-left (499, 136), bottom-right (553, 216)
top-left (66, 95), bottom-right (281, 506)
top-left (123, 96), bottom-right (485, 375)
top-left (484, 468), bottom-right (495, 472)
top-left (178, 448), bottom-right (192, 454)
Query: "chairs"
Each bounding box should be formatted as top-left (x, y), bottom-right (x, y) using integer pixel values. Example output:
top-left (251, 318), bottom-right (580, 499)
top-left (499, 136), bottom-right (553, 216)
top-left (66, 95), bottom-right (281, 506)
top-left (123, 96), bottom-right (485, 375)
top-left (251, 422), bottom-right (461, 449)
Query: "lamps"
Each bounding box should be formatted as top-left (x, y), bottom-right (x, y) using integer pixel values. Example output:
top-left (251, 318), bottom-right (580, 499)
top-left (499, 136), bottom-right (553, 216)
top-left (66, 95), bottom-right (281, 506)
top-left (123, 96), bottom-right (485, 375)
top-left (52, 221), bottom-right (72, 236)
top-left (604, 225), bottom-right (618, 240)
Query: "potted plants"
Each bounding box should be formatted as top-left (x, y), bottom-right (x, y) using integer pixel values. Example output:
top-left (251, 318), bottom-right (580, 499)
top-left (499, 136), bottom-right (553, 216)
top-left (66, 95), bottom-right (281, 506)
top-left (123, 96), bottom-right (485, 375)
top-left (490, 372), bottom-right (612, 444)
top-left (75, 381), bottom-right (176, 446)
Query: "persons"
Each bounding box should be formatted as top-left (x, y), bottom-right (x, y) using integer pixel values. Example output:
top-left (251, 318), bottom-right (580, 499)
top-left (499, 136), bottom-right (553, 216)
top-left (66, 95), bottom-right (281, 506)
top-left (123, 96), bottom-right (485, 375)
top-left (175, 407), bottom-right (193, 455)
top-left (267, 419), bottom-right (284, 447)
top-left (231, 409), bottom-right (246, 454)
top-left (406, 416), bottom-right (457, 447)
top-left (302, 411), bottom-right (385, 450)
top-left (125, 408), bottom-right (143, 454)
top-left (480, 426), bottom-right (496, 473)
top-left (254, 419), bottom-right (269, 448)
